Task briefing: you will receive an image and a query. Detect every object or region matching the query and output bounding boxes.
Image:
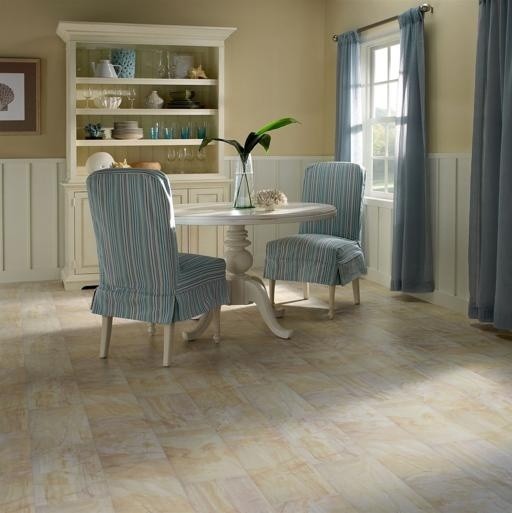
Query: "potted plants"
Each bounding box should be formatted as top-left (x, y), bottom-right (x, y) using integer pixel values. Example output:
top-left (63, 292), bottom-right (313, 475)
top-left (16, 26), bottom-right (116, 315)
top-left (198, 115), bottom-right (302, 210)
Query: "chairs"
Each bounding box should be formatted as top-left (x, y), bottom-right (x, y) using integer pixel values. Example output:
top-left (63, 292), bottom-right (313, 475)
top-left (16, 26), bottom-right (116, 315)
top-left (264, 161), bottom-right (368, 319)
top-left (85, 167), bottom-right (227, 367)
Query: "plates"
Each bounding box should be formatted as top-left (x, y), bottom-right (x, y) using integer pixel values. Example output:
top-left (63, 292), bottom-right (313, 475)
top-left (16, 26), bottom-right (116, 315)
top-left (111, 120), bottom-right (144, 139)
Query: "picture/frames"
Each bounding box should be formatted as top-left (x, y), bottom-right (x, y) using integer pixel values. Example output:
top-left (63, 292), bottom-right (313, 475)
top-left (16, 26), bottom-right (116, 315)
top-left (0, 57), bottom-right (41, 137)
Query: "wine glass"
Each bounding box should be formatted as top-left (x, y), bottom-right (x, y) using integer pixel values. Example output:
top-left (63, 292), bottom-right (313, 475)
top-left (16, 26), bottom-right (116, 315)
top-left (83, 86), bottom-right (138, 108)
top-left (166, 147), bottom-right (207, 173)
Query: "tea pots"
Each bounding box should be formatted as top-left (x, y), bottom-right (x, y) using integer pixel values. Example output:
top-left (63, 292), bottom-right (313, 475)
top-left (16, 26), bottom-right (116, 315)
top-left (88, 59), bottom-right (123, 77)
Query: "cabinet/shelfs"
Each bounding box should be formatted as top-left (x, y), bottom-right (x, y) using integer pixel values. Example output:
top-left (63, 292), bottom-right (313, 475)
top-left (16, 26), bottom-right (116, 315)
top-left (58, 180), bottom-right (233, 292)
top-left (56, 21), bottom-right (238, 183)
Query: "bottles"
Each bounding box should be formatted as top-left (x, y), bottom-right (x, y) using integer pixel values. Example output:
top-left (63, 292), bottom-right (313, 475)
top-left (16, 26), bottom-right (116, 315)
top-left (144, 90), bottom-right (164, 107)
top-left (154, 46), bottom-right (177, 79)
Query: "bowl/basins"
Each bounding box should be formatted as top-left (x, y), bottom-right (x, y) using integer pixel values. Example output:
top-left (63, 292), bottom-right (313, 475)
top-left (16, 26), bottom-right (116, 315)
top-left (130, 161), bottom-right (161, 170)
top-left (168, 89), bottom-right (195, 99)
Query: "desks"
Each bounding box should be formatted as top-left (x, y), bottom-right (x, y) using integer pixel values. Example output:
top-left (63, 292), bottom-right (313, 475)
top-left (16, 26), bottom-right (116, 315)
top-left (172, 202), bottom-right (338, 343)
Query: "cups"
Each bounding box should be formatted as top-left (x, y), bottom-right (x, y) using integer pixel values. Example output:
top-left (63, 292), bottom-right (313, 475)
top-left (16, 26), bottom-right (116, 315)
top-left (150, 119), bottom-right (206, 138)
